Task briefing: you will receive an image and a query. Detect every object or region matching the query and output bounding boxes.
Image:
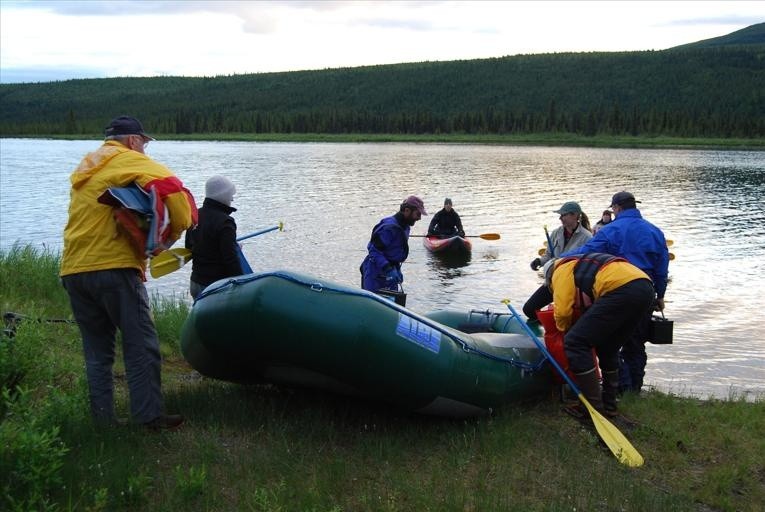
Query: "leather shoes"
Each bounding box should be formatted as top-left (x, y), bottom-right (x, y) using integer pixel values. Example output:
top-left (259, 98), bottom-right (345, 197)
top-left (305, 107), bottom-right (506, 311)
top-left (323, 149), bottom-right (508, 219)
top-left (144, 414), bottom-right (185, 431)
top-left (111, 417), bottom-right (130, 426)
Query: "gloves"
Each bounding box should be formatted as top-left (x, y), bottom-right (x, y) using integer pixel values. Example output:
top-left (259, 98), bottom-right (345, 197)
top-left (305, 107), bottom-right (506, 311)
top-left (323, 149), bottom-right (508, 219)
top-left (429, 231), bottom-right (433, 235)
top-left (457, 230), bottom-right (465, 238)
top-left (531, 257), bottom-right (542, 270)
top-left (654, 298), bottom-right (664, 311)
top-left (386, 269), bottom-right (403, 283)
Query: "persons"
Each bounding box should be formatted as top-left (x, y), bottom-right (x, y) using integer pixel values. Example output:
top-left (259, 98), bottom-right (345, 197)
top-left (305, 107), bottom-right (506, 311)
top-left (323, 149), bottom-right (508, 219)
top-left (520, 201), bottom-right (595, 319)
top-left (541, 252), bottom-right (657, 417)
top-left (427, 197), bottom-right (465, 241)
top-left (58, 115), bottom-right (201, 432)
top-left (556, 191), bottom-right (668, 397)
top-left (183, 173), bottom-right (244, 306)
top-left (590, 210), bottom-right (613, 237)
top-left (358, 196), bottom-right (428, 302)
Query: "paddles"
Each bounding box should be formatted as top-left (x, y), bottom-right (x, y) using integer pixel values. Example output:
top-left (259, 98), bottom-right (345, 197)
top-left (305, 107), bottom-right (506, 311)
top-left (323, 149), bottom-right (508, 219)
top-left (538, 249), bottom-right (547, 256)
top-left (148, 223), bottom-right (284, 280)
top-left (668, 252), bottom-right (675, 261)
top-left (409, 233), bottom-right (501, 241)
top-left (502, 299), bottom-right (644, 470)
top-left (543, 241), bottom-right (549, 247)
top-left (665, 240), bottom-right (674, 246)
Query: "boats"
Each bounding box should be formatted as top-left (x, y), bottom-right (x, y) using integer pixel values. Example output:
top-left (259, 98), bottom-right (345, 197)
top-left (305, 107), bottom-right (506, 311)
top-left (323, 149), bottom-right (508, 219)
top-left (423, 235), bottom-right (472, 256)
top-left (179, 270), bottom-right (546, 415)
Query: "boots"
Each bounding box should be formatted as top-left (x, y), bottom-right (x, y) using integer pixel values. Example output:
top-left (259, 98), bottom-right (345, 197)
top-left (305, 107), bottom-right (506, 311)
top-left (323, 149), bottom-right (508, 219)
top-left (574, 367), bottom-right (620, 425)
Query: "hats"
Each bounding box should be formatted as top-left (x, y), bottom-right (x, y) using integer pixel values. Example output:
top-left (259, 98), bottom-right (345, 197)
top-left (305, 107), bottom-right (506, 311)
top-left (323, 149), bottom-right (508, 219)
top-left (445, 198), bottom-right (451, 205)
top-left (405, 196), bottom-right (427, 216)
top-left (206, 176), bottom-right (237, 204)
top-left (609, 191), bottom-right (635, 207)
top-left (106, 116), bottom-right (156, 141)
top-left (553, 202), bottom-right (581, 215)
top-left (544, 258), bottom-right (558, 286)
